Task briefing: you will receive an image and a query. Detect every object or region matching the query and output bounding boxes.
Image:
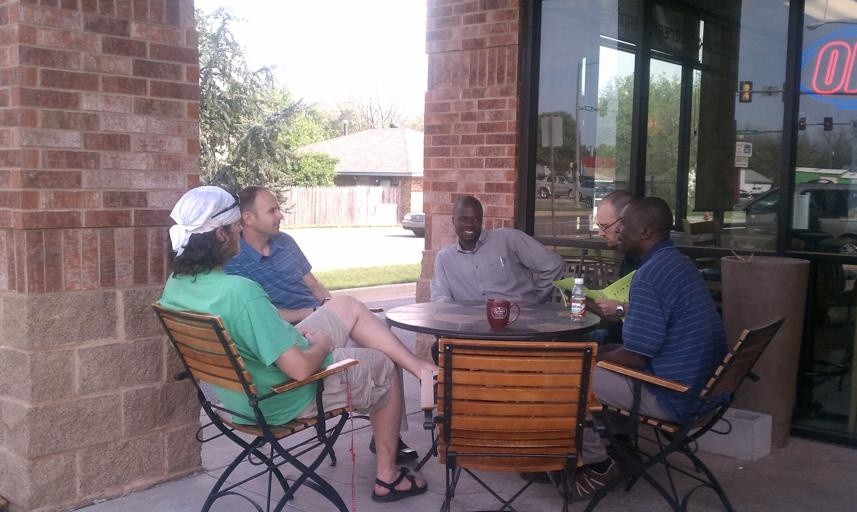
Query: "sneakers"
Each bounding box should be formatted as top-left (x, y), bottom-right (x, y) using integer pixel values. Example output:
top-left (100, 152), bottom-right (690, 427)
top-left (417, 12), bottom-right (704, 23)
top-left (560, 457), bottom-right (621, 499)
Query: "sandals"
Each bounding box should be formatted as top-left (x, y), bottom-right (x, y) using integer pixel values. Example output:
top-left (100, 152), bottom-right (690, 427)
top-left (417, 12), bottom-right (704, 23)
top-left (371, 468), bottom-right (429, 500)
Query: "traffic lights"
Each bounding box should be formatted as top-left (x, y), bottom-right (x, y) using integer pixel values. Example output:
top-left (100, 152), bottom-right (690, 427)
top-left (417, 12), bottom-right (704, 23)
top-left (799, 114), bottom-right (807, 129)
top-left (823, 117), bottom-right (833, 133)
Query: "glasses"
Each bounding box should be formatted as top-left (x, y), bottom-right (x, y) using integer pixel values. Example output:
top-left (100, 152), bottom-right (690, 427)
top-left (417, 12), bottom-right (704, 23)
top-left (597, 219), bottom-right (621, 230)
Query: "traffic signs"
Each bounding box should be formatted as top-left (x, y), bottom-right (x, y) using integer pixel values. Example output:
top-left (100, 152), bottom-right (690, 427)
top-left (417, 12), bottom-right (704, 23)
top-left (582, 104), bottom-right (594, 111)
top-left (737, 128), bottom-right (758, 135)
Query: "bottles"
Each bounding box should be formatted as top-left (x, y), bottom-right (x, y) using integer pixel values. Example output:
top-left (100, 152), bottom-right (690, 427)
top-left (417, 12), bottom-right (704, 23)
top-left (568, 279), bottom-right (590, 324)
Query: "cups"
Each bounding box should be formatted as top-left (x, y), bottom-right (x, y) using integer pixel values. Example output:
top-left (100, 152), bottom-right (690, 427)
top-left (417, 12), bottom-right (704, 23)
top-left (486, 298), bottom-right (521, 329)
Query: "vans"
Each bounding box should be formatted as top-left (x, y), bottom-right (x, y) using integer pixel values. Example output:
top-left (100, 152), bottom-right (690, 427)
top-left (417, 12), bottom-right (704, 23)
top-left (690, 180), bottom-right (857, 259)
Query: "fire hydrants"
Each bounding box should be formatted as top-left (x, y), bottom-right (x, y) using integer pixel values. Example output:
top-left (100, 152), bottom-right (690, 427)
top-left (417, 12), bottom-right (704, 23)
top-left (701, 211), bottom-right (711, 223)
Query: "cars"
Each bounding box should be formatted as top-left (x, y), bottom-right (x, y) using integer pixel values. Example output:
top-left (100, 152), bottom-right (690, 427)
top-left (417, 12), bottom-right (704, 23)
top-left (399, 211), bottom-right (426, 240)
top-left (537, 169), bottom-right (625, 219)
top-left (735, 192), bottom-right (757, 209)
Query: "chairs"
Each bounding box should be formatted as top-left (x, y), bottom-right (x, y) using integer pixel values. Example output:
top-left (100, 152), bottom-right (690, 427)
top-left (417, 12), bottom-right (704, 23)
top-left (368, 296), bottom-right (386, 320)
top-left (420, 340), bottom-right (594, 511)
top-left (592, 318), bottom-right (785, 512)
top-left (151, 304), bottom-right (359, 511)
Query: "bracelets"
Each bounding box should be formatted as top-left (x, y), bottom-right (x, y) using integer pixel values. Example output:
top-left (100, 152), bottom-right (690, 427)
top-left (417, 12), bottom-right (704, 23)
top-left (312, 305), bottom-right (316, 312)
top-left (319, 296), bottom-right (334, 306)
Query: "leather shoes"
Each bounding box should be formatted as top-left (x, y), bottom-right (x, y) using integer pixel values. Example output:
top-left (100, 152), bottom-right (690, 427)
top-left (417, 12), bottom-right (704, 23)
top-left (368, 432), bottom-right (420, 463)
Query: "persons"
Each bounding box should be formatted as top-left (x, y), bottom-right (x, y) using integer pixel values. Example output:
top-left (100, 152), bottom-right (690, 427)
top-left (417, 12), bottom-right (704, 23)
top-left (557, 196), bottom-right (734, 501)
top-left (762, 187), bottom-right (846, 420)
top-left (220, 186), bottom-right (421, 462)
top-left (157, 184), bottom-right (438, 502)
top-left (429, 194), bottom-right (569, 303)
top-left (517, 188), bottom-right (644, 482)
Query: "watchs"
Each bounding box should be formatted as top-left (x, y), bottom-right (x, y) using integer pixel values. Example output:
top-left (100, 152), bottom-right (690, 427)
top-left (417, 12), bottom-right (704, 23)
top-left (614, 301), bottom-right (627, 317)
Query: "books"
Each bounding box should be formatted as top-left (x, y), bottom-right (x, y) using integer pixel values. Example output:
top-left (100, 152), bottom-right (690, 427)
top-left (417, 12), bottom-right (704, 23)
top-left (549, 269), bottom-right (637, 309)
